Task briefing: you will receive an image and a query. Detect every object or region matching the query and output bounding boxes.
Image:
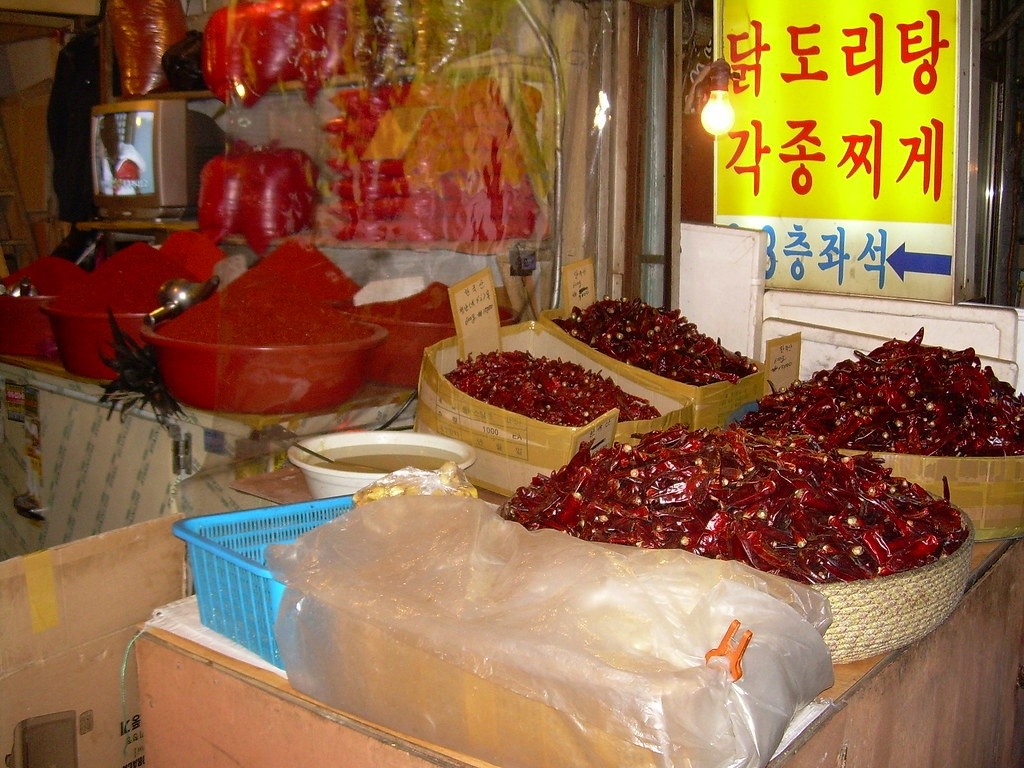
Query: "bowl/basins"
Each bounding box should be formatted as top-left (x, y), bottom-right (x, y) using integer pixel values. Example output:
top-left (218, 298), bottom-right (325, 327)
top-left (288, 430), bottom-right (477, 500)
top-left (0, 290), bottom-right (518, 416)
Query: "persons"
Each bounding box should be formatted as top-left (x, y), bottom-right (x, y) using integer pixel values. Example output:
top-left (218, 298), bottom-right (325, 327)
top-left (46, 0), bottom-right (107, 270)
top-left (99, 112), bottom-right (145, 196)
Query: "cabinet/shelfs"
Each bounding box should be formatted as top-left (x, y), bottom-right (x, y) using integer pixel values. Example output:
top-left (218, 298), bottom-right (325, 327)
top-left (75, 53), bottom-right (556, 316)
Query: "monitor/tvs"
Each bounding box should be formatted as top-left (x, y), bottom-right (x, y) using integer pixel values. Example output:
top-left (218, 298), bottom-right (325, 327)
top-left (87, 99), bottom-right (230, 222)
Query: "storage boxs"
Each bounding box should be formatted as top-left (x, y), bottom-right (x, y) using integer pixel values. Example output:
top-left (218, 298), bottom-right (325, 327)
top-left (0, 513), bottom-right (189, 768)
top-left (539, 304), bottom-right (762, 431)
top-left (414, 318), bottom-right (693, 497)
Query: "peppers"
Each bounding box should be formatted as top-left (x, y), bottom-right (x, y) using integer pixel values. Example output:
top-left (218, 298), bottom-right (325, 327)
top-left (738, 325), bottom-right (1024, 458)
top-left (499, 422), bottom-right (969, 587)
top-left (552, 296), bottom-right (758, 388)
top-left (440, 349), bottom-right (662, 429)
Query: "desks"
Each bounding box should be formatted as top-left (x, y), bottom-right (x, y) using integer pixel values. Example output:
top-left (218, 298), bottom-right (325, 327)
top-left (132, 535), bottom-right (1024, 768)
top-left (0, 351), bottom-right (418, 554)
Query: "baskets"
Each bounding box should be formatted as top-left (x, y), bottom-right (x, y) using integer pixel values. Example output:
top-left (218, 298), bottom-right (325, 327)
top-left (805, 490), bottom-right (974, 662)
top-left (171, 493), bottom-right (358, 671)
